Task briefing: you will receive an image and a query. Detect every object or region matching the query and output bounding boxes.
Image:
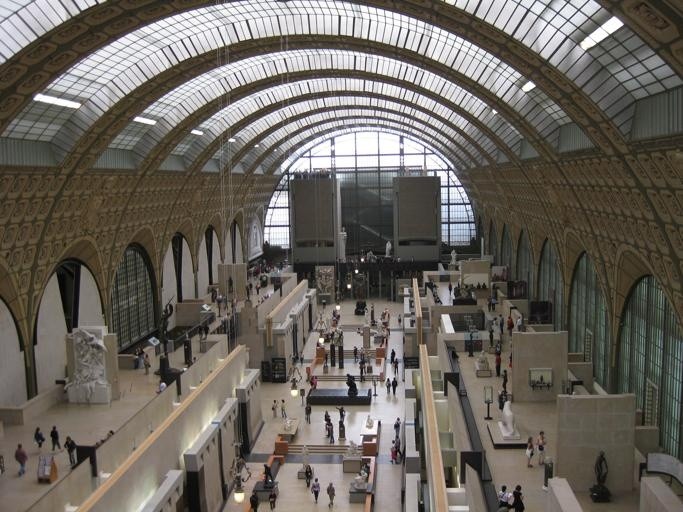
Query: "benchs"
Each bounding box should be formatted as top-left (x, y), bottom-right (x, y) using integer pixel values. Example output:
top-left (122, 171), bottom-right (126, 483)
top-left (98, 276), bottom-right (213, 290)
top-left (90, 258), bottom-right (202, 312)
top-left (447, 345), bottom-right (503, 512)
top-left (268, 459), bottom-right (282, 482)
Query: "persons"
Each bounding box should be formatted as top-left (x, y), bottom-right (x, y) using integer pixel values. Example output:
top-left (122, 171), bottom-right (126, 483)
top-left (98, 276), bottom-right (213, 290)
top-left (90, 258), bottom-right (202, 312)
top-left (263, 464), bottom-right (274, 483)
top-left (64, 436), bottom-right (77, 467)
top-left (50, 425), bottom-right (62, 452)
top-left (273, 399), bottom-right (287, 417)
top-left (143, 354), bottom-right (150, 374)
top-left (305, 403), bottom-right (311, 424)
top-left (247, 260), bottom-right (283, 294)
top-left (298, 308), bottom-right (402, 394)
top-left (450, 250), bottom-right (457, 265)
top-left (14, 443), bottom-right (28, 477)
top-left (497, 485), bottom-right (525, 511)
top-left (385, 241), bottom-right (391, 256)
top-left (594, 451), bottom-right (608, 485)
top-left (448, 273), bottom-right (522, 359)
top-left (269, 490), bottom-right (276, 510)
top-left (527, 431), bottom-right (546, 468)
top-left (390, 417), bottom-right (402, 463)
top-left (204, 324), bottom-right (209, 336)
top-left (493, 353), bottom-right (513, 413)
top-left (325, 406), bottom-right (345, 443)
top-left (311, 478), bottom-right (320, 502)
top-left (327, 482), bottom-right (336, 507)
top-left (249, 491), bottom-right (258, 511)
top-left (33, 426), bottom-right (45, 453)
top-left (356, 470), bottom-right (368, 485)
top-left (305, 465), bottom-right (312, 488)
